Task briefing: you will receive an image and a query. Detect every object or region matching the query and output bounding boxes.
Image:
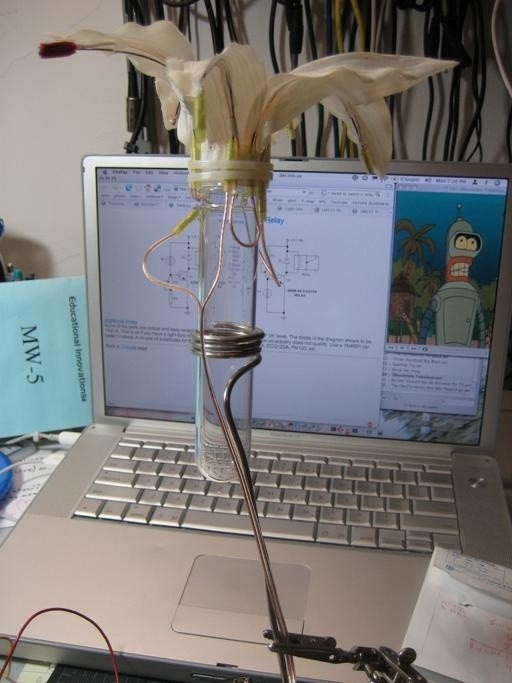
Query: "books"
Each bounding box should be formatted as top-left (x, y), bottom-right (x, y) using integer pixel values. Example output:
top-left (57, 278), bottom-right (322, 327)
top-left (0, 275), bottom-right (93, 529)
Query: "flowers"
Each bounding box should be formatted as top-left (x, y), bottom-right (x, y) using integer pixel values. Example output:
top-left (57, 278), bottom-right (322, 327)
top-left (35, 19), bottom-right (465, 232)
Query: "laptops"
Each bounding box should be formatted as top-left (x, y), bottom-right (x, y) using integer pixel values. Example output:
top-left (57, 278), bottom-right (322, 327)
top-left (0, 155), bottom-right (512, 683)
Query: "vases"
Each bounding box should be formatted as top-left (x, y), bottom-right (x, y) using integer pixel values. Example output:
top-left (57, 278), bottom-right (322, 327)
top-left (182, 160), bottom-right (273, 484)
